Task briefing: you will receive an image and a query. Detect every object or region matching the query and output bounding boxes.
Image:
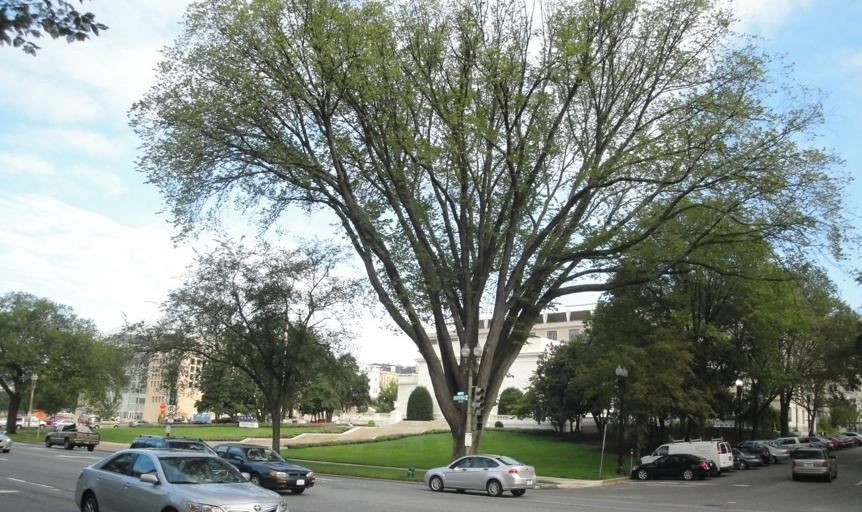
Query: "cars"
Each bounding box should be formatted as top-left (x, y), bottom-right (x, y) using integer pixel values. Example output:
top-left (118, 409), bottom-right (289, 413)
top-left (424, 454), bottom-right (537, 496)
top-left (631, 432), bottom-right (862, 482)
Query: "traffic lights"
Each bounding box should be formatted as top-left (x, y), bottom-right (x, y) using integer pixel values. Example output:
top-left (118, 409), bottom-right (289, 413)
top-left (472, 386), bottom-right (484, 431)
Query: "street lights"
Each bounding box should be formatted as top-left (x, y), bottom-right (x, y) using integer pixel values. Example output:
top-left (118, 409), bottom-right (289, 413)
top-left (735, 376), bottom-right (743, 440)
top-left (461, 343), bottom-right (485, 455)
top-left (616, 361), bottom-right (628, 474)
top-left (27, 373), bottom-right (39, 428)
